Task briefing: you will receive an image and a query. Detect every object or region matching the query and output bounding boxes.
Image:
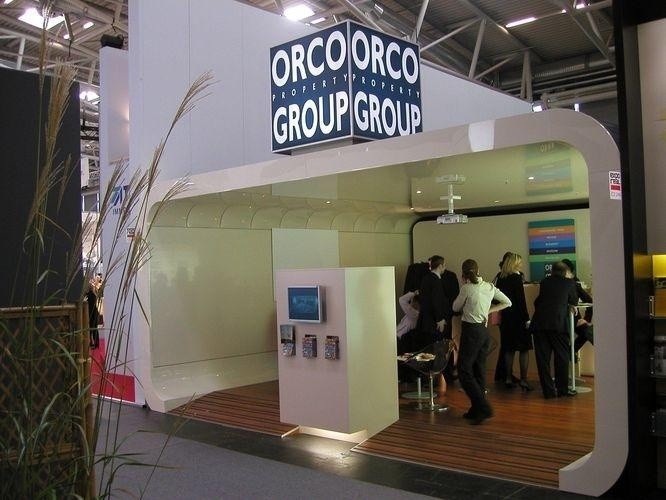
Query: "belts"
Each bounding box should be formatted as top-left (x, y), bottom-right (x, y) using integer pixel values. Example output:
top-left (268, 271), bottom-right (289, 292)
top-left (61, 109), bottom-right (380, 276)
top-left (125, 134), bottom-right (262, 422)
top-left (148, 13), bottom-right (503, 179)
top-left (461, 321), bottom-right (486, 327)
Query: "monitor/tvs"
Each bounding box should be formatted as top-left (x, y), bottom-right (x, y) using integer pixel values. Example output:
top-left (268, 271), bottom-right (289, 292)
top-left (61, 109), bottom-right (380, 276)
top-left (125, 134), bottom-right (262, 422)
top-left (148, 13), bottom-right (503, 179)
top-left (287, 285), bottom-right (322, 322)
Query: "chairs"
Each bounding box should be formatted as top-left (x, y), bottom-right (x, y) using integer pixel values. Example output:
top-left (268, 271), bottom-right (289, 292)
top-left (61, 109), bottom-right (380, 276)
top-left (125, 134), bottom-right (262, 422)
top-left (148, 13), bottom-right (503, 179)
top-left (397, 340), bottom-right (455, 415)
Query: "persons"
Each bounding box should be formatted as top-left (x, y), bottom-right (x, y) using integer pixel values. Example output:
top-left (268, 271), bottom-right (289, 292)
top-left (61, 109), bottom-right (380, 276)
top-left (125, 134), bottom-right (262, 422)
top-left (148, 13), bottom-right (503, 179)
top-left (415, 254), bottom-right (458, 387)
top-left (84, 282), bottom-right (101, 350)
top-left (491, 252), bottom-right (523, 384)
top-left (426, 257), bottom-right (461, 374)
top-left (88, 272), bottom-right (103, 294)
top-left (452, 258), bottom-right (513, 424)
top-left (500, 252), bottom-right (535, 393)
top-left (561, 259), bottom-right (593, 364)
top-left (529, 261), bottom-right (580, 399)
top-left (396, 288), bottom-right (421, 385)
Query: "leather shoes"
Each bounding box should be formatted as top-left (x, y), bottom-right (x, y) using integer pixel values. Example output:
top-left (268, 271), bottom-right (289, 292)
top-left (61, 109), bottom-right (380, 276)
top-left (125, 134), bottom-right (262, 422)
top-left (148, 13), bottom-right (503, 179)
top-left (403, 373), bottom-right (577, 399)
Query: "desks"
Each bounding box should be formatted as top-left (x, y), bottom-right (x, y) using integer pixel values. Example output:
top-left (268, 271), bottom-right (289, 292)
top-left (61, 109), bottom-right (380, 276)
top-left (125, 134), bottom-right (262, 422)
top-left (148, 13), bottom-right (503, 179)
top-left (565, 303), bottom-right (593, 394)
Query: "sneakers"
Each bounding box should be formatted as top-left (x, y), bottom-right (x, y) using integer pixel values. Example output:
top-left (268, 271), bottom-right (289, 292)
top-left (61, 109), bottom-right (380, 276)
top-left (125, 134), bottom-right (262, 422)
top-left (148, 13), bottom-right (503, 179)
top-left (463, 409), bottom-right (496, 425)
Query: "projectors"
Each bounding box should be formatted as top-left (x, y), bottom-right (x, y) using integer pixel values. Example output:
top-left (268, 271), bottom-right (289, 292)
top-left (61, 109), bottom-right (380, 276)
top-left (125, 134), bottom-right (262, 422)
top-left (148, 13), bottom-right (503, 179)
top-left (437, 214), bottom-right (468, 224)
